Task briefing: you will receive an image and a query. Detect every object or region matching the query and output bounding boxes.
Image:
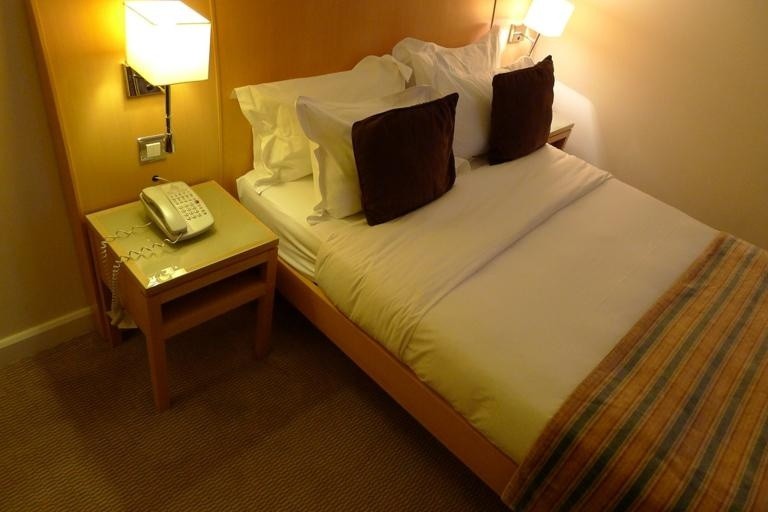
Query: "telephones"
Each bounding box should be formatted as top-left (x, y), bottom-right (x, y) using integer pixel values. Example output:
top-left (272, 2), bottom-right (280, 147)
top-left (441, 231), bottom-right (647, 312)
top-left (139, 180), bottom-right (214, 242)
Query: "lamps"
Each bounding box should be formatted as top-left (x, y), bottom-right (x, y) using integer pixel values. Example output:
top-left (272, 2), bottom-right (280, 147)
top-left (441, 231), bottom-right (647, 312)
top-left (506, 0), bottom-right (576, 59)
top-left (120, 1), bottom-right (210, 161)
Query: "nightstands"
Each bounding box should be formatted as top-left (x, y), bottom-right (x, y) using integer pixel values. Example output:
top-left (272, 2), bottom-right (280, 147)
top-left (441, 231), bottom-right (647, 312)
top-left (545, 107), bottom-right (574, 152)
top-left (88, 177), bottom-right (277, 412)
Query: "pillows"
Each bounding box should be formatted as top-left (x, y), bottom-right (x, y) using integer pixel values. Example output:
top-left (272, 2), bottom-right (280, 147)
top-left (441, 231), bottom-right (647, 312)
top-left (224, 55), bottom-right (416, 195)
top-left (388, 21), bottom-right (505, 84)
top-left (353, 94), bottom-right (458, 226)
top-left (486, 51), bottom-right (554, 163)
top-left (407, 53), bottom-right (537, 162)
top-left (291, 84), bottom-right (439, 227)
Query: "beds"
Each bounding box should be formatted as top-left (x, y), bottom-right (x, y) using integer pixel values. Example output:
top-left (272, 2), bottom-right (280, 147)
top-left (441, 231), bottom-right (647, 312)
top-left (216, 0), bottom-right (768, 512)
